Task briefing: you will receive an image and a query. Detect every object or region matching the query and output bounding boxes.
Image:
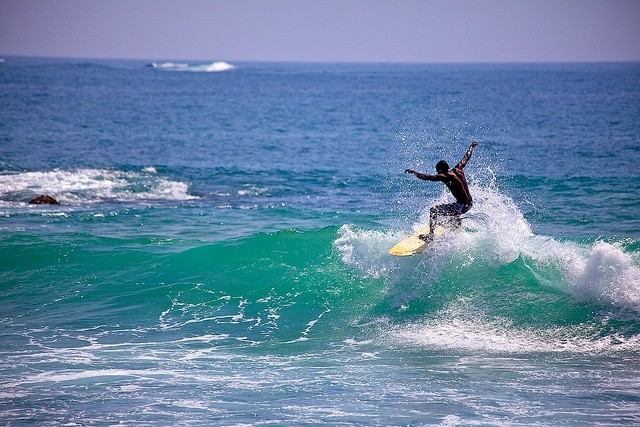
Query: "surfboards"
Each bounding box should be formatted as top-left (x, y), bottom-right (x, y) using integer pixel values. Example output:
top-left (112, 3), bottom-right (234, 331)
top-left (389, 223), bottom-right (447, 256)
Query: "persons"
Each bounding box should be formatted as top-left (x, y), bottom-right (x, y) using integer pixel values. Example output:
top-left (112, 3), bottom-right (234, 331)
top-left (404, 139), bottom-right (477, 242)
